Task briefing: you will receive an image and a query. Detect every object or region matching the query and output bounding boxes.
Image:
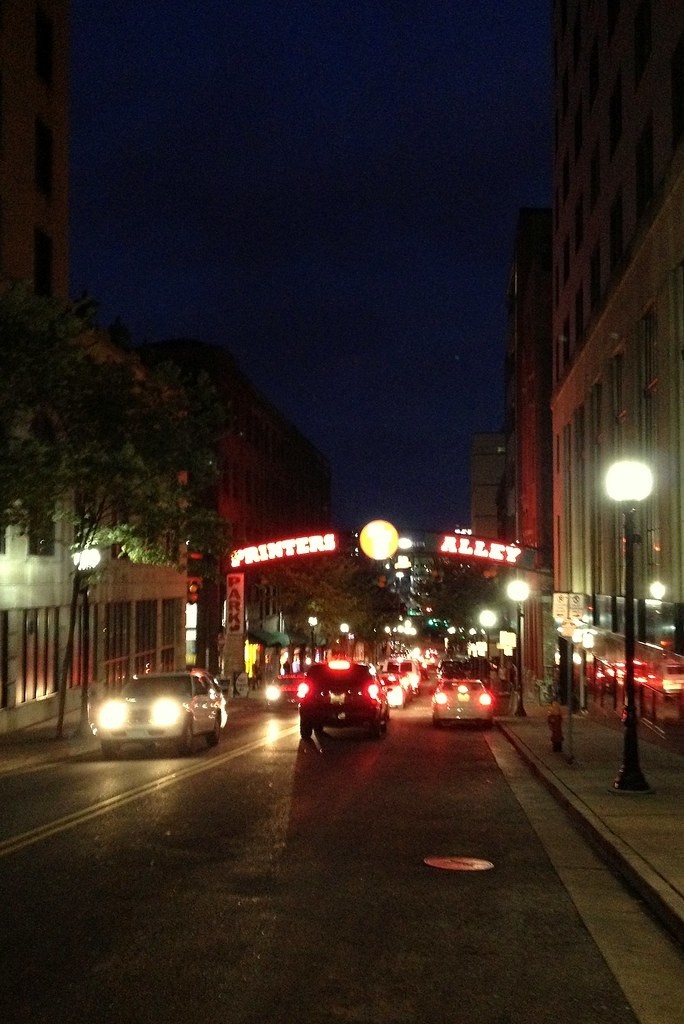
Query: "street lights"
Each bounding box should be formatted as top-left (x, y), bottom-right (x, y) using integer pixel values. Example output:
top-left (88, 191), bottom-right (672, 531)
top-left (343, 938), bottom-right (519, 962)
top-left (71, 547), bottom-right (102, 738)
top-left (507, 579), bottom-right (531, 717)
top-left (479, 609), bottom-right (498, 687)
top-left (606, 460), bottom-right (656, 795)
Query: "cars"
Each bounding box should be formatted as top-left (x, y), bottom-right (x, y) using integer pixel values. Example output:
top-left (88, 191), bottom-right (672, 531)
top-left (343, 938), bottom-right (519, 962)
top-left (266, 672), bottom-right (305, 711)
top-left (96, 670), bottom-right (228, 760)
top-left (378, 655), bottom-right (439, 709)
top-left (431, 679), bottom-right (495, 728)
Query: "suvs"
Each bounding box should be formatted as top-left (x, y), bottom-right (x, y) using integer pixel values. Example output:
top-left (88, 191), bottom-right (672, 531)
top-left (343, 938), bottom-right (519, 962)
top-left (297, 659), bottom-right (393, 738)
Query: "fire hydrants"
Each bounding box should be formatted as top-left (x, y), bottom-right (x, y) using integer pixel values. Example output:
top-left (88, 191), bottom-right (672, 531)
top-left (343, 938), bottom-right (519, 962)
top-left (547, 702), bottom-right (565, 753)
